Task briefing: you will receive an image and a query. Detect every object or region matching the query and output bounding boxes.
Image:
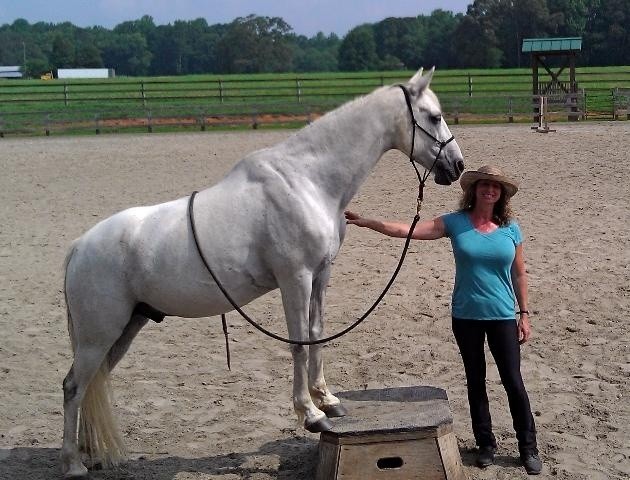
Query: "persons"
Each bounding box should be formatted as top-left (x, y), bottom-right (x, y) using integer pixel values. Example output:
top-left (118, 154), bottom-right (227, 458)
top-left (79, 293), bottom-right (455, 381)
top-left (343, 166), bottom-right (542, 474)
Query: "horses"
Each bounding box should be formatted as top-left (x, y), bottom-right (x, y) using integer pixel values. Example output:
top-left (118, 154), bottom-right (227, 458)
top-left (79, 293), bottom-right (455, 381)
top-left (60, 68), bottom-right (465, 479)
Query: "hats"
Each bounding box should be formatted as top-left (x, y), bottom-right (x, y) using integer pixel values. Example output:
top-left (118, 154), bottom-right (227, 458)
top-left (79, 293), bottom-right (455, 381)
top-left (460, 164), bottom-right (518, 197)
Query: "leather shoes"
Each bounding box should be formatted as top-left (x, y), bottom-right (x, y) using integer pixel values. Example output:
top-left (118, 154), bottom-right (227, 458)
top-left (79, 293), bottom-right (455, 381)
top-left (476, 444), bottom-right (498, 467)
top-left (520, 453), bottom-right (542, 475)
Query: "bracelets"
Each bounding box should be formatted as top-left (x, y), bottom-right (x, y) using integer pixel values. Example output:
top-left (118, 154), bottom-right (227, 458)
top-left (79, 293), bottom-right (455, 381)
top-left (516, 310), bottom-right (529, 315)
